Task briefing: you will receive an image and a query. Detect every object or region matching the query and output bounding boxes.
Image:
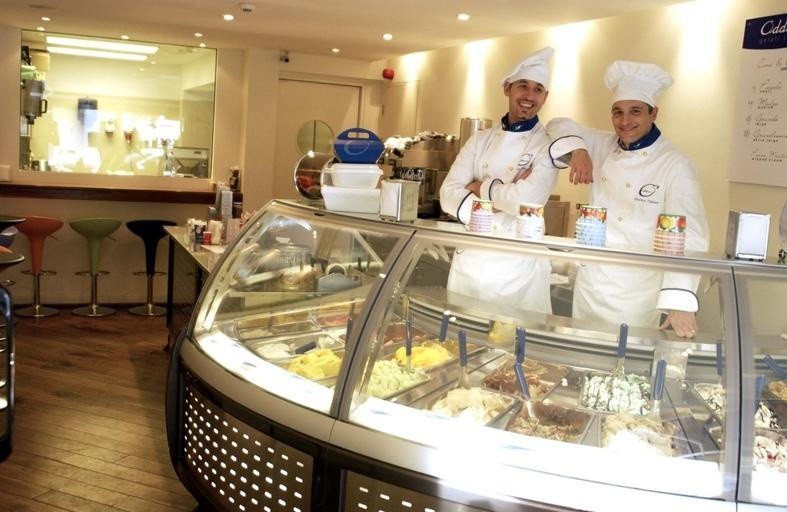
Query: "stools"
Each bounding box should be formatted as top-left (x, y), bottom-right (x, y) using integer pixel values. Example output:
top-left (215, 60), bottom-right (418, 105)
top-left (0, 216), bottom-right (26, 237)
top-left (0, 226), bottom-right (18, 247)
top-left (69, 218), bottom-right (121, 317)
top-left (1, 245), bottom-right (26, 461)
top-left (126, 220), bottom-right (177, 317)
top-left (14, 217), bottom-right (64, 318)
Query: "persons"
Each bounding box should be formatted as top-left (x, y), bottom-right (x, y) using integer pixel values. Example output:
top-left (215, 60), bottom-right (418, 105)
top-left (439, 47), bottom-right (560, 315)
top-left (545, 60), bottom-right (710, 338)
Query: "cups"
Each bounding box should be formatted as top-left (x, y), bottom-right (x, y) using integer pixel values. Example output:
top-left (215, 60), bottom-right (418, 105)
top-left (574, 203), bottom-right (607, 247)
top-left (652, 213), bottom-right (687, 256)
top-left (516, 202), bottom-right (546, 236)
top-left (469, 199), bottom-right (494, 232)
top-left (194, 224), bottom-right (206, 244)
top-left (202, 232), bottom-right (212, 243)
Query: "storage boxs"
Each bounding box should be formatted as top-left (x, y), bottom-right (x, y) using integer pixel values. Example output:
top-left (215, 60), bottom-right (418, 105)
top-left (335, 128), bottom-right (385, 164)
top-left (329, 164), bottom-right (380, 190)
top-left (320, 185), bottom-right (382, 214)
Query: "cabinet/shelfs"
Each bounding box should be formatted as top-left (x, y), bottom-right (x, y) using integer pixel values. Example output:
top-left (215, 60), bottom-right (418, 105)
top-left (163, 200), bottom-right (786, 511)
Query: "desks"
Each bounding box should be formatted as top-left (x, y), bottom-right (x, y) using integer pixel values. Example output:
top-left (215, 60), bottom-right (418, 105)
top-left (163, 225), bottom-right (232, 328)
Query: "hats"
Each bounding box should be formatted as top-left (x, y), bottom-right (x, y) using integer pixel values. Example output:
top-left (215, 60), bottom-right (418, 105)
top-left (604, 60), bottom-right (673, 111)
top-left (502, 47), bottom-right (555, 93)
